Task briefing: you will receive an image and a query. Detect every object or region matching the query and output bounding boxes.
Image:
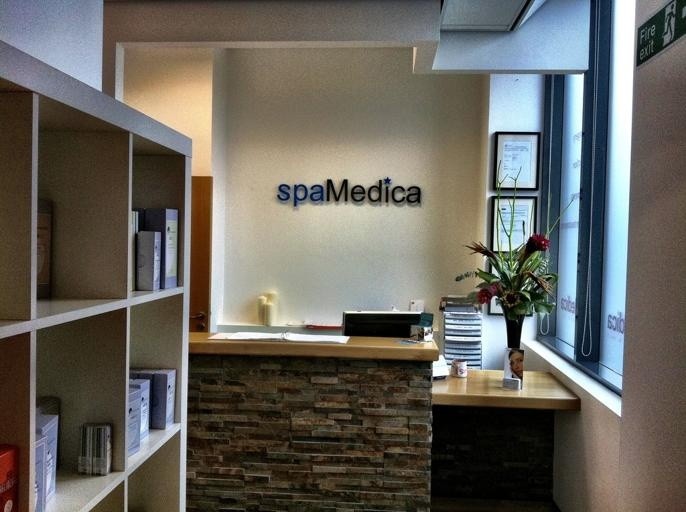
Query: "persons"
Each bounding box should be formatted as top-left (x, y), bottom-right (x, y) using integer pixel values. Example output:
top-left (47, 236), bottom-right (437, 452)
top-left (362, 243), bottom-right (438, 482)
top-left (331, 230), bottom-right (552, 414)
top-left (509, 348), bottom-right (523, 382)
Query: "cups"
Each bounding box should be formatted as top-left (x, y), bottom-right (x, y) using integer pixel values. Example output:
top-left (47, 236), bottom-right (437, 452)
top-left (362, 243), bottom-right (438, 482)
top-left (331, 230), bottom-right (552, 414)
top-left (451, 358), bottom-right (467, 378)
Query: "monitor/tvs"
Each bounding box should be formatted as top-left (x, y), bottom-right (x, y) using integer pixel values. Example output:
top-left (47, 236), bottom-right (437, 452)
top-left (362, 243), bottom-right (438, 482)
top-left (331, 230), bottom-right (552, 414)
top-left (343, 311), bottom-right (422, 339)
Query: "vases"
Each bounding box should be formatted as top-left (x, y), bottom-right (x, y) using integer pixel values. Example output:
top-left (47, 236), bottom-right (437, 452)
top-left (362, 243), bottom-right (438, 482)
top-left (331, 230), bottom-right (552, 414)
top-left (500, 304), bottom-right (528, 348)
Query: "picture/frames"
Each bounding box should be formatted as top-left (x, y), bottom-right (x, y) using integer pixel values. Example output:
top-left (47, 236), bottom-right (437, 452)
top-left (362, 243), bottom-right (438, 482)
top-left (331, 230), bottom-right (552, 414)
top-left (488, 130), bottom-right (542, 317)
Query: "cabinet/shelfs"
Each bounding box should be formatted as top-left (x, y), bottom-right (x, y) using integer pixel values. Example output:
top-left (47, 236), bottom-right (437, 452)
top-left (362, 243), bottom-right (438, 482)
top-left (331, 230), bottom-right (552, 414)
top-left (0, 35), bottom-right (193, 512)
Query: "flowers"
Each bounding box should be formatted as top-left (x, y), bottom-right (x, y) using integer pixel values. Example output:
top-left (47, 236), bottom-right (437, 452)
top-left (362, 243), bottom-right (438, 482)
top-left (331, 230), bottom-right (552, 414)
top-left (452, 161), bottom-right (580, 317)
